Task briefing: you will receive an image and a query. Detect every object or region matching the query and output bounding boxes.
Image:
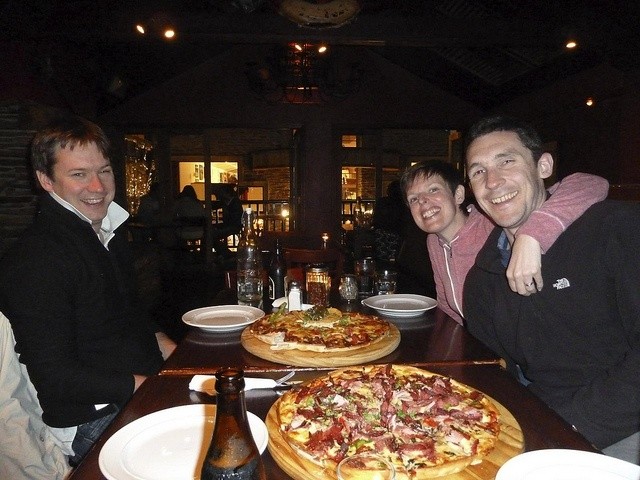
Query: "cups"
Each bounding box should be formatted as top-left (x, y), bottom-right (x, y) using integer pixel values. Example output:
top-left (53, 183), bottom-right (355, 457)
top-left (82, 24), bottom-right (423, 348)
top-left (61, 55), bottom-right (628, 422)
top-left (375, 264), bottom-right (397, 294)
top-left (356, 255), bottom-right (375, 296)
top-left (339, 274), bottom-right (359, 310)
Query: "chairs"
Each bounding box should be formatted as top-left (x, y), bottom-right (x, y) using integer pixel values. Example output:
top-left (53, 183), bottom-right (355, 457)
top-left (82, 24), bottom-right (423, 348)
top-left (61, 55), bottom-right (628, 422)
top-left (279, 246), bottom-right (345, 285)
top-left (172, 216), bottom-right (205, 256)
top-left (220, 210), bottom-right (248, 250)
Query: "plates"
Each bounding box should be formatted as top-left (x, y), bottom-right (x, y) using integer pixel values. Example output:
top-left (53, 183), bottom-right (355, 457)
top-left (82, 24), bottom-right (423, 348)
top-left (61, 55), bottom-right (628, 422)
top-left (98, 403), bottom-right (269, 479)
top-left (493, 448), bottom-right (639, 480)
top-left (182, 304), bottom-right (265, 333)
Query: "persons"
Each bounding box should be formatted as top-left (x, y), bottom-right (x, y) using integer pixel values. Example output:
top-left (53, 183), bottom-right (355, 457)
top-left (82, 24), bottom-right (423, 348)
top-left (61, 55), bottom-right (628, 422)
top-left (461, 110), bottom-right (640, 464)
top-left (135, 182), bottom-right (162, 214)
top-left (0, 108), bottom-right (177, 466)
top-left (402, 158), bottom-right (610, 325)
top-left (170, 185), bottom-right (207, 233)
top-left (0, 309), bottom-right (72, 480)
top-left (349, 196), bottom-right (389, 236)
top-left (358, 174), bottom-right (407, 268)
top-left (211, 185), bottom-right (244, 264)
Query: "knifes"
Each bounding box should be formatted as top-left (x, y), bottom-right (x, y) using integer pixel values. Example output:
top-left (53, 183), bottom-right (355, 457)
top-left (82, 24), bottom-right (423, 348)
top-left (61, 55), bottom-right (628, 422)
top-left (274, 379), bottom-right (303, 387)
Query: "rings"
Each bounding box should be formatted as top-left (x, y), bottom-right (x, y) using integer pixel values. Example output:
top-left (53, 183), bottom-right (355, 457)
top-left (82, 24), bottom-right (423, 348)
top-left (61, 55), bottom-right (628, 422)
top-left (524, 281), bottom-right (534, 287)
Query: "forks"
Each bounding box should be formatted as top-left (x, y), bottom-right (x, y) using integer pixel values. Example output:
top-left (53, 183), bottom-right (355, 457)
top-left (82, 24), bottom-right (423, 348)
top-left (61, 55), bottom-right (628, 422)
top-left (272, 370), bottom-right (295, 383)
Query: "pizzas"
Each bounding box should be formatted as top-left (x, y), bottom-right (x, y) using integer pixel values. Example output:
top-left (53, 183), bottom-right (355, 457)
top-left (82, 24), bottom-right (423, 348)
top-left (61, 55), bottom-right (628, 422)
top-left (278, 364), bottom-right (500, 479)
top-left (251, 307), bottom-right (390, 352)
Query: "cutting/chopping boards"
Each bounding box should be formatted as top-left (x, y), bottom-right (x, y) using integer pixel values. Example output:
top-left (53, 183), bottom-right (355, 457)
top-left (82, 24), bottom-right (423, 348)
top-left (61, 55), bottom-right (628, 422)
top-left (241, 320), bottom-right (402, 367)
top-left (265, 364), bottom-right (526, 480)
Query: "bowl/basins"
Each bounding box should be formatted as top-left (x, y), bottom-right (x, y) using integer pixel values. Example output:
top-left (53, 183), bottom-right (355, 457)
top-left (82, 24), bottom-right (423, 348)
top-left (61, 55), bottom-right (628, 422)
top-left (363, 293), bottom-right (439, 317)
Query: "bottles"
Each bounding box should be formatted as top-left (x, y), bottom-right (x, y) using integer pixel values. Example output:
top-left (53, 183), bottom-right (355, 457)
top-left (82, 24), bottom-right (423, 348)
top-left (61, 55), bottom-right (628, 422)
top-left (288, 280), bottom-right (303, 310)
top-left (304, 263), bottom-right (332, 308)
top-left (236, 208), bottom-right (264, 310)
top-left (200, 365), bottom-right (266, 480)
top-left (352, 199), bottom-right (362, 229)
top-left (269, 237), bottom-right (285, 301)
